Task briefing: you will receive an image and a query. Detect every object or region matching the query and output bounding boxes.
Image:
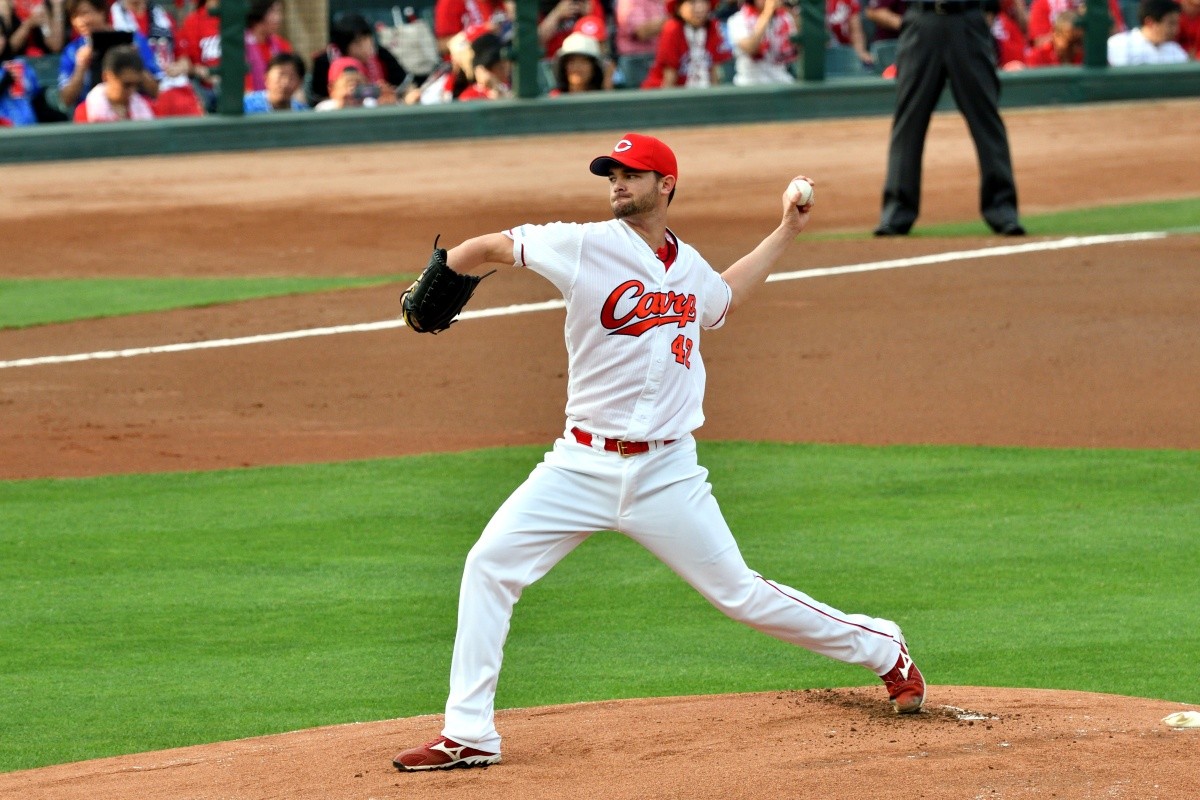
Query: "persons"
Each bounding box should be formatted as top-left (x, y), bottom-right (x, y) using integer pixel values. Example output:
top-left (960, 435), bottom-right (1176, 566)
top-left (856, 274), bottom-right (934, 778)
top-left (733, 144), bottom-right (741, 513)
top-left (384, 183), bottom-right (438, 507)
top-left (0, 0), bottom-right (1200, 127)
top-left (393, 132), bottom-right (928, 773)
top-left (862, 0), bottom-right (1030, 239)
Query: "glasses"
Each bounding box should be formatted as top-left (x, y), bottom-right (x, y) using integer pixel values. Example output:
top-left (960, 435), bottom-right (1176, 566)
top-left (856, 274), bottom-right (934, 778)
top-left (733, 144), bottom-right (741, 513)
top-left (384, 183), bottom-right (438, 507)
top-left (112, 71), bottom-right (139, 89)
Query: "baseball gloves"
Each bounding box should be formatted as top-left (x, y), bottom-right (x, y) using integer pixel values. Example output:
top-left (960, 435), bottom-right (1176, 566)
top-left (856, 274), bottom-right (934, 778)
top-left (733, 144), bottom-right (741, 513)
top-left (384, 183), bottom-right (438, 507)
top-left (399, 233), bottom-right (496, 335)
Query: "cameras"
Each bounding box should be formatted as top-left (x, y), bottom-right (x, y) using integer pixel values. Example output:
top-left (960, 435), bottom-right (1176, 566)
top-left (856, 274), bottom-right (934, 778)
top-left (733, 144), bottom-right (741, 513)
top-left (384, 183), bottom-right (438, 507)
top-left (353, 84), bottom-right (382, 99)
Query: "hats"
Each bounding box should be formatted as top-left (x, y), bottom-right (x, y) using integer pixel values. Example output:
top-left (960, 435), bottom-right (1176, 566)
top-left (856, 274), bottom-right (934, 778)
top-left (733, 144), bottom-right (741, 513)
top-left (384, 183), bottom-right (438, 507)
top-left (328, 56), bottom-right (369, 86)
top-left (557, 32), bottom-right (603, 62)
top-left (589, 133), bottom-right (678, 184)
top-left (573, 15), bottom-right (607, 42)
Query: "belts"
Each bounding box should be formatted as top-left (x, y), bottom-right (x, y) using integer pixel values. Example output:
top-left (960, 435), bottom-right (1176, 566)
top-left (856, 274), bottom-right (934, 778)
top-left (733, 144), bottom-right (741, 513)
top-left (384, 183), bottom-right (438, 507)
top-left (571, 428), bottom-right (675, 457)
top-left (910, 1), bottom-right (985, 15)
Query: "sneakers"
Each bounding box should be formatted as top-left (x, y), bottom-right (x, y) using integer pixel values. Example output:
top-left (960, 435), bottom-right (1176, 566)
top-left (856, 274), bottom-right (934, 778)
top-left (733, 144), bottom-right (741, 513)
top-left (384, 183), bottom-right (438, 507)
top-left (392, 736), bottom-right (502, 771)
top-left (879, 623), bottom-right (926, 714)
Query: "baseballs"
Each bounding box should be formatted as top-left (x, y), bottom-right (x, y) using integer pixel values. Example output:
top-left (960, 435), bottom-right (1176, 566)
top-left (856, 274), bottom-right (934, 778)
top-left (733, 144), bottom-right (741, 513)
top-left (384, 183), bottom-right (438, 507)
top-left (787, 179), bottom-right (813, 206)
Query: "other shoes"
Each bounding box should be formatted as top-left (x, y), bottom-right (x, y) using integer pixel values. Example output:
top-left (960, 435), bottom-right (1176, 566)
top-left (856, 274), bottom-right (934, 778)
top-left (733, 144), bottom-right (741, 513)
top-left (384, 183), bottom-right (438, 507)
top-left (874, 226), bottom-right (908, 236)
top-left (1001, 222), bottom-right (1025, 235)
